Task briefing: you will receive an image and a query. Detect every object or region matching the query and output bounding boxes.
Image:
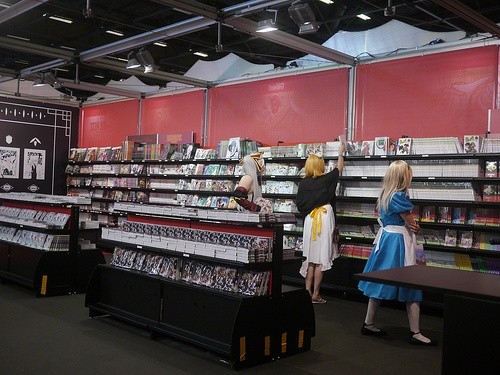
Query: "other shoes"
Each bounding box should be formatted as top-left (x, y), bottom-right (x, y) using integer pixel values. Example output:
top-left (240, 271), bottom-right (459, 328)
top-left (361, 322), bottom-right (387, 337)
top-left (409, 331), bottom-right (438, 345)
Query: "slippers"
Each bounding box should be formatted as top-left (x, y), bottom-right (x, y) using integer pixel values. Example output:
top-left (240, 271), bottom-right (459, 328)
top-left (312, 297), bottom-right (326, 303)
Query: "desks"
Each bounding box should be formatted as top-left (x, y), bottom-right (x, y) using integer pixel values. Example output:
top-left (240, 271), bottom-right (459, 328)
top-left (353, 264), bottom-right (500, 375)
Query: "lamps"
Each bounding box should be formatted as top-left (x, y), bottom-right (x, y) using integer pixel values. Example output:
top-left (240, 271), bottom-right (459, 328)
top-left (256, 9), bottom-right (279, 33)
top-left (288, 0), bottom-right (323, 36)
top-left (136, 48), bottom-right (160, 74)
top-left (126, 51), bottom-right (141, 70)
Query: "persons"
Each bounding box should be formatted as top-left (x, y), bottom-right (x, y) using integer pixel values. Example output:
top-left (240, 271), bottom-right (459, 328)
top-left (294, 142), bottom-right (346, 304)
top-left (234, 153), bottom-right (265, 212)
top-left (358, 160), bottom-right (437, 346)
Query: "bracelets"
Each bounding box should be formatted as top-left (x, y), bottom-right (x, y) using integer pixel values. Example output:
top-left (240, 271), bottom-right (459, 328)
top-left (338, 154), bottom-right (343, 157)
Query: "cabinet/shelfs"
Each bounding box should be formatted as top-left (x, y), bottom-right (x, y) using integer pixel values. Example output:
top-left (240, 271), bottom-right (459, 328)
top-left (0, 152), bottom-right (500, 375)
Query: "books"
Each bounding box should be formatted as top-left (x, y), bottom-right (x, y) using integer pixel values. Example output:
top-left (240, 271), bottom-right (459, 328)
top-left (3, 133), bottom-right (499, 296)
